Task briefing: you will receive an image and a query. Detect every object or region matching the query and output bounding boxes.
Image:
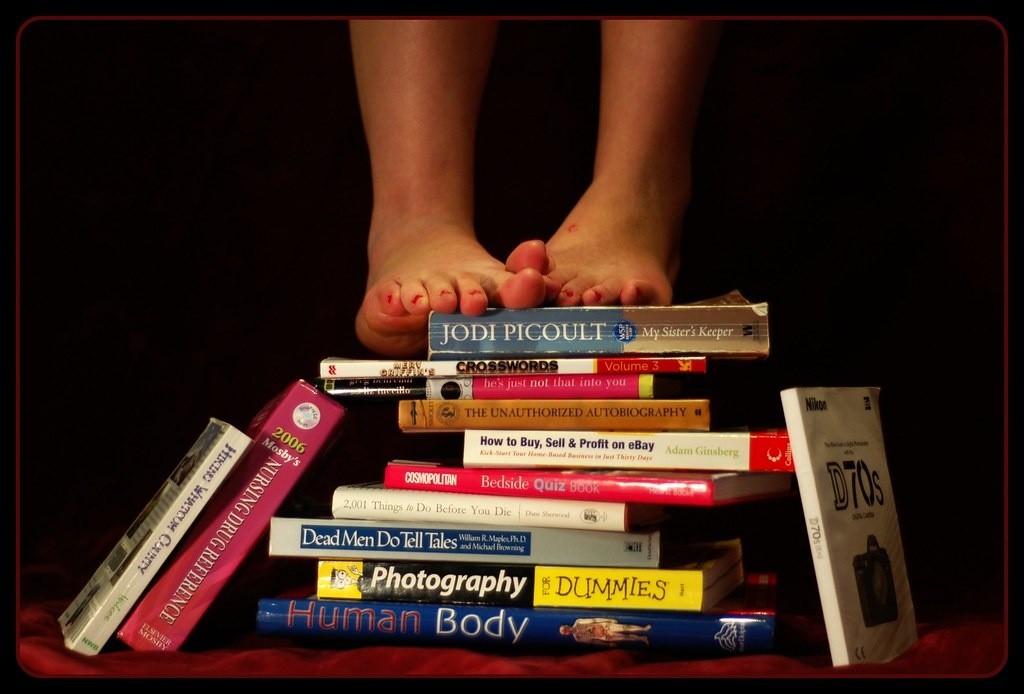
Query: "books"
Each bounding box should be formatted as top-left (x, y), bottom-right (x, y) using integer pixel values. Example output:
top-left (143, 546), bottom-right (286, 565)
top-left (256, 291), bottom-right (795, 665)
top-left (781, 386), bottom-right (917, 666)
top-left (59, 379), bottom-right (345, 656)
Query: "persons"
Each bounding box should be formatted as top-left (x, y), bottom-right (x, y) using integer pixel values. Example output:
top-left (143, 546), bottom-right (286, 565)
top-left (355, 20), bottom-right (697, 355)
